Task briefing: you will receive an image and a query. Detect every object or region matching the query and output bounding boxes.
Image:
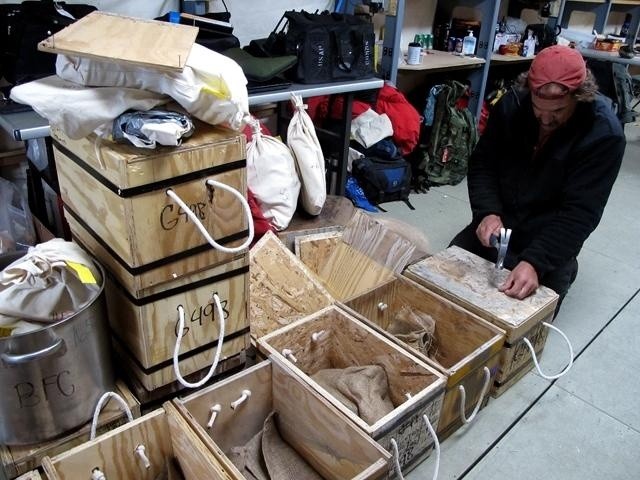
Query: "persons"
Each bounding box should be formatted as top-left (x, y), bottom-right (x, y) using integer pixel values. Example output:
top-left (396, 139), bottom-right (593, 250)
top-left (447, 44), bottom-right (626, 320)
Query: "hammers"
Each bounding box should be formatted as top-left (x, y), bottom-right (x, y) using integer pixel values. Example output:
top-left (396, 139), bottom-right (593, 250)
top-left (489, 228), bottom-right (513, 271)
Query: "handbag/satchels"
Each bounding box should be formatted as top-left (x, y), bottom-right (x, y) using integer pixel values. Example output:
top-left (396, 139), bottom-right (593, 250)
top-left (152, 11), bottom-right (243, 54)
top-left (110, 105), bottom-right (196, 151)
top-left (54, 33), bottom-right (253, 136)
top-left (352, 153), bottom-right (417, 212)
top-left (243, 114), bottom-right (301, 232)
top-left (0, 234), bottom-right (106, 331)
top-left (286, 93), bottom-right (329, 219)
top-left (300, 84), bottom-right (420, 161)
top-left (280, 7), bottom-right (375, 85)
top-left (7, 71), bottom-right (170, 144)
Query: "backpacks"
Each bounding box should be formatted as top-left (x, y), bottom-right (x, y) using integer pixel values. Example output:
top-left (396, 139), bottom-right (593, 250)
top-left (421, 81), bottom-right (481, 188)
top-left (522, 22), bottom-right (563, 57)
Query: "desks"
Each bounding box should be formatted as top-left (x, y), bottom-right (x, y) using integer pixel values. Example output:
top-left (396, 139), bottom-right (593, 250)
top-left (0, 75), bottom-right (386, 242)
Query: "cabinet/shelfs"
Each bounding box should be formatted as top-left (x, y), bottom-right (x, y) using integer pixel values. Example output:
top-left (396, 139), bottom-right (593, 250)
top-left (346, 0), bottom-right (638, 129)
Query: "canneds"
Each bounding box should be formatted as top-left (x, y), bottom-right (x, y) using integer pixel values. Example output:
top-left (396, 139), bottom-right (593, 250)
top-left (446, 36), bottom-right (463, 53)
top-left (416, 33), bottom-right (434, 50)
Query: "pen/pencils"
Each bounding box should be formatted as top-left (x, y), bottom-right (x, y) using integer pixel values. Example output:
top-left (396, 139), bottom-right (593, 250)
top-left (593, 30), bottom-right (600, 39)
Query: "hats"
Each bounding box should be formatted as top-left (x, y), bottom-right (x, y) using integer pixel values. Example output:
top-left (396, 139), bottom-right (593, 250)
top-left (527, 43), bottom-right (590, 101)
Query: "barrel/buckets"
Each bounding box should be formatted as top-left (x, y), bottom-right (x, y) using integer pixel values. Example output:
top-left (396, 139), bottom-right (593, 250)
top-left (0, 249), bottom-right (115, 448)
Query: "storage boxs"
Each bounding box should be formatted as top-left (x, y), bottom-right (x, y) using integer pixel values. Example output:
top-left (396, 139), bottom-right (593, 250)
top-left (402, 245), bottom-right (559, 397)
top-left (335, 275), bottom-right (506, 450)
top-left (51, 124), bottom-right (255, 290)
top-left (0, 196), bottom-right (447, 479)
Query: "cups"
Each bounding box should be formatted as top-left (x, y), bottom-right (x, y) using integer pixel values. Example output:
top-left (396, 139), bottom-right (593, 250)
top-left (406, 42), bottom-right (422, 64)
